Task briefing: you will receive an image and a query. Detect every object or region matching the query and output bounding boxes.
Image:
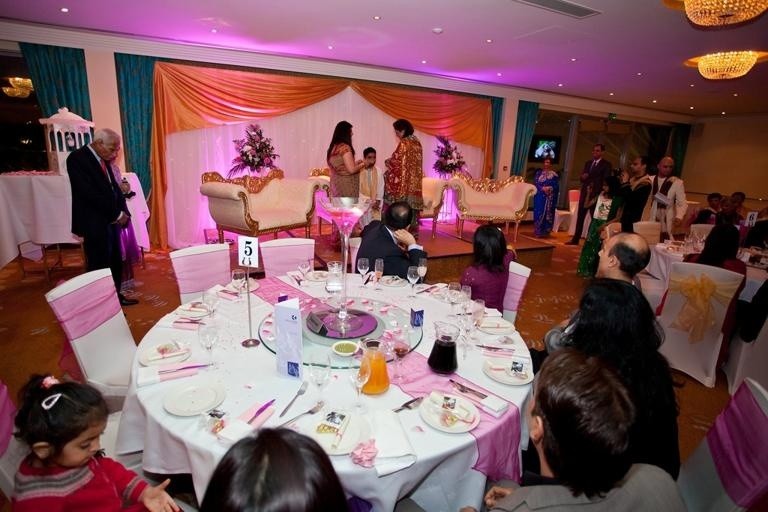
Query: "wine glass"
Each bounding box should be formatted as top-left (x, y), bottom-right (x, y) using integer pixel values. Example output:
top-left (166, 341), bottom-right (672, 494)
top-left (308, 196), bottom-right (378, 340)
top-left (448, 282), bottom-right (462, 304)
top-left (417, 258), bottom-right (427, 284)
top-left (350, 355), bottom-right (372, 413)
top-left (232, 269), bottom-right (246, 295)
top-left (358, 258), bottom-right (369, 286)
top-left (461, 285), bottom-right (471, 315)
top-left (198, 320), bottom-right (219, 369)
top-left (407, 265), bottom-right (419, 293)
top-left (202, 290), bottom-right (219, 318)
top-left (374, 258), bottom-right (384, 283)
top-left (308, 353), bottom-right (332, 400)
top-left (297, 262), bottom-right (310, 276)
top-left (391, 331), bottom-right (410, 384)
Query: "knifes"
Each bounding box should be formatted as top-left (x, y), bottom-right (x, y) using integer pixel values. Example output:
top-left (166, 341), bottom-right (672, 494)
top-left (477, 343), bottom-right (516, 354)
top-left (247, 398), bottom-right (275, 425)
top-left (158, 364), bottom-right (207, 374)
top-left (448, 379), bottom-right (487, 400)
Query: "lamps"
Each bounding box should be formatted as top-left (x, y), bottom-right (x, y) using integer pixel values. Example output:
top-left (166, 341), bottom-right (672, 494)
top-left (663, 0), bottom-right (768, 27)
top-left (685, 51), bottom-right (766, 81)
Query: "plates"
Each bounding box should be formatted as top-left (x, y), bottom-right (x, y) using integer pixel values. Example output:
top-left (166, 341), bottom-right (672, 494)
top-left (478, 317), bottom-right (515, 336)
top-left (227, 281), bottom-right (259, 293)
top-left (420, 394), bottom-right (481, 433)
top-left (304, 408), bottom-right (368, 457)
top-left (306, 270), bottom-right (328, 282)
top-left (163, 382), bottom-right (225, 417)
top-left (483, 357), bottom-right (534, 386)
top-left (182, 307), bottom-right (206, 317)
top-left (382, 275), bottom-right (408, 287)
top-left (139, 341), bottom-right (192, 367)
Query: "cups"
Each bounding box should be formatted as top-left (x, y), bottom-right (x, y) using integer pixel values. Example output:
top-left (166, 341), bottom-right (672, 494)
top-left (328, 260), bottom-right (342, 283)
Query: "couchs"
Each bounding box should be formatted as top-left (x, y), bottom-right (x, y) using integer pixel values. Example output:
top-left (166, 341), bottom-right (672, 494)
top-left (200, 168), bottom-right (321, 240)
top-left (309, 165), bottom-right (450, 250)
top-left (449, 172), bottom-right (537, 242)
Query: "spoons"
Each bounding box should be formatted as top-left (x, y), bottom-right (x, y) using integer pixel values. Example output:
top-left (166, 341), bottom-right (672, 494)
top-left (393, 395), bottom-right (422, 415)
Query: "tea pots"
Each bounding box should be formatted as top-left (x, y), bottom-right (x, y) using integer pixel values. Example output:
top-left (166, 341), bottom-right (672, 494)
top-left (426, 320), bottom-right (460, 376)
top-left (362, 337), bottom-right (392, 395)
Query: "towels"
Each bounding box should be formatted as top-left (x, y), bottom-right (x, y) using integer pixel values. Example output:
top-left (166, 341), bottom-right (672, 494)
top-left (181, 299), bottom-right (215, 314)
top-left (459, 306), bottom-right (502, 319)
top-left (483, 343), bottom-right (531, 361)
top-left (322, 407), bottom-right (418, 478)
top-left (135, 359), bottom-right (211, 389)
top-left (362, 270), bottom-right (376, 286)
top-left (449, 381), bottom-right (510, 419)
top-left (285, 269), bottom-right (307, 290)
top-left (207, 283), bottom-right (241, 302)
top-left (215, 398), bottom-right (280, 446)
top-left (418, 280), bottom-right (449, 296)
top-left (156, 313), bottom-right (202, 332)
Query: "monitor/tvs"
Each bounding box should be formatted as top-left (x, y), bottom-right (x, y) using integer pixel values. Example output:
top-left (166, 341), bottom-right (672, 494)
top-left (528, 135), bottom-right (563, 164)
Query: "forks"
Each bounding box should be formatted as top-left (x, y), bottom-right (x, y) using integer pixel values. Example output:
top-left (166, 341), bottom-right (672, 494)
top-left (277, 402), bottom-right (325, 429)
top-left (280, 380), bottom-right (308, 418)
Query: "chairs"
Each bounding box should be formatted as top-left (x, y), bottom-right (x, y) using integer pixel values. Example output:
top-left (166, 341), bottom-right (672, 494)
top-left (606, 222), bottom-right (621, 240)
top-left (721, 317), bottom-right (768, 397)
top-left (677, 376), bottom-right (768, 512)
top-left (656, 259), bottom-right (745, 388)
top-left (503, 260), bottom-right (531, 324)
top-left (259, 237), bottom-right (315, 279)
top-left (569, 190), bottom-right (580, 214)
top-left (633, 221), bottom-right (661, 244)
top-left (169, 243), bottom-right (230, 305)
top-left (349, 237), bottom-right (361, 274)
top-left (45, 267), bottom-right (137, 393)
top-left (0, 383), bottom-right (151, 499)
top-left (691, 224), bottom-right (715, 242)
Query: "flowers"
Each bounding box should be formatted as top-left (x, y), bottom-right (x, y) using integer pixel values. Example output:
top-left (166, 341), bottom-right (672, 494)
top-left (225, 122), bottom-right (280, 179)
top-left (432, 133), bottom-right (466, 178)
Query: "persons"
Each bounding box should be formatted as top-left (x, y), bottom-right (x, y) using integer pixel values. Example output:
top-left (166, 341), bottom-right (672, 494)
top-left (389, 349), bottom-right (688, 512)
top-left (383, 120), bottom-right (424, 254)
top-left (66, 128), bottom-right (139, 307)
top-left (200, 427), bottom-right (353, 512)
top-left (461, 225), bottom-right (517, 317)
top-left (8, 374), bottom-right (186, 512)
top-left (686, 187), bottom-right (768, 266)
top-left (525, 230), bottom-right (650, 372)
top-left (354, 202), bottom-right (429, 284)
top-left (325, 121), bottom-right (374, 252)
top-left (564, 143), bottom-right (611, 246)
top-left (647, 155), bottom-right (688, 246)
top-left (519, 276), bottom-right (682, 486)
top-left (575, 176), bottom-right (623, 281)
top-left (618, 156), bottom-right (653, 234)
top-left (532, 157), bottom-right (559, 237)
top-left (357, 146), bottom-right (377, 205)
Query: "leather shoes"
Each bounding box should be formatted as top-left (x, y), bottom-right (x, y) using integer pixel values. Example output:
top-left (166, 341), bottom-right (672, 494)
top-left (120, 295), bottom-right (141, 307)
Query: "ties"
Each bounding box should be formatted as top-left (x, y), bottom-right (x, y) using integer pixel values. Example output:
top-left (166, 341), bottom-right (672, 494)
top-left (99, 157), bottom-right (108, 178)
top-left (588, 160), bottom-right (597, 175)
top-left (367, 167), bottom-right (372, 189)
